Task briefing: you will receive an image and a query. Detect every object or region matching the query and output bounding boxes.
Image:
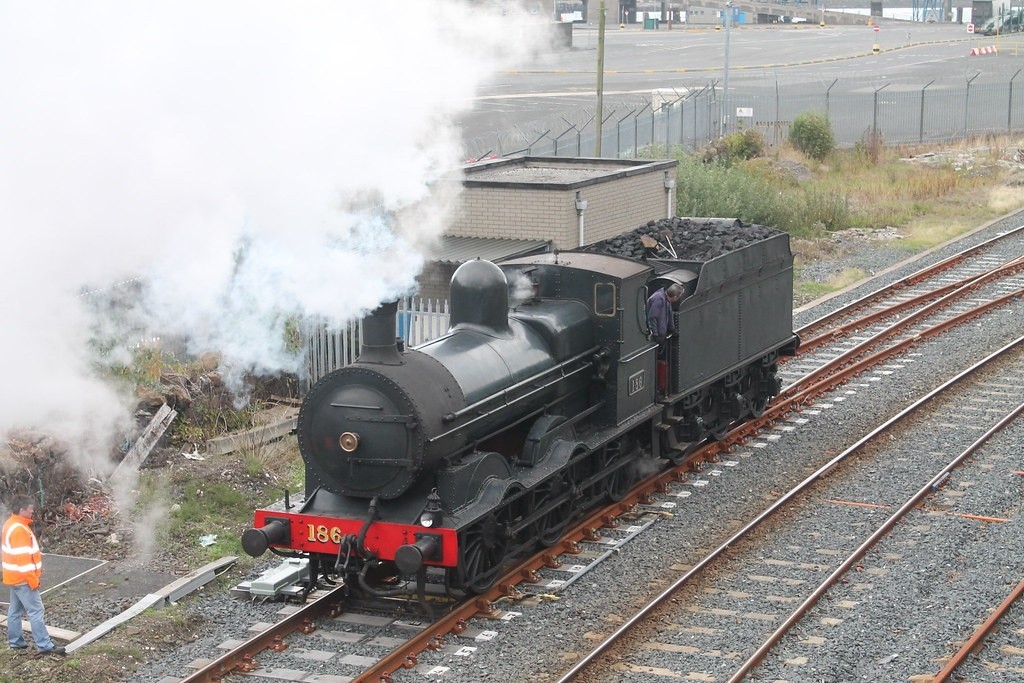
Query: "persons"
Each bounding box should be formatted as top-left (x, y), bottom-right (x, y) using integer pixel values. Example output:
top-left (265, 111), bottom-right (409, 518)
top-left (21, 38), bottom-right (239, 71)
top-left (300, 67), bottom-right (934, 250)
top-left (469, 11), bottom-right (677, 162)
top-left (648, 284), bottom-right (685, 345)
top-left (2, 494), bottom-right (66, 654)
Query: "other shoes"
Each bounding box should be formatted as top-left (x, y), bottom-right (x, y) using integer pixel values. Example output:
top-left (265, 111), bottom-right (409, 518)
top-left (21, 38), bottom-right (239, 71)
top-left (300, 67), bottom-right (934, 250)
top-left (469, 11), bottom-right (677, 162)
top-left (20, 645), bottom-right (28, 649)
top-left (47, 645), bottom-right (65, 653)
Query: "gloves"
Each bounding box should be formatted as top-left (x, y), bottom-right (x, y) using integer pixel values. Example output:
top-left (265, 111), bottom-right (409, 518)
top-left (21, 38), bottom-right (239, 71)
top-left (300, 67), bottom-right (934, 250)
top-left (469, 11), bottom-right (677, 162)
top-left (670, 329), bottom-right (679, 337)
top-left (655, 336), bottom-right (665, 346)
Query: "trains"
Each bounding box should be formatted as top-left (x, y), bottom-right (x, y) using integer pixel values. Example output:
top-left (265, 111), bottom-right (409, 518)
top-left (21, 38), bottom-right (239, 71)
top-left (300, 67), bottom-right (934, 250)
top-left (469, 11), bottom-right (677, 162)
top-left (240, 216), bottom-right (803, 602)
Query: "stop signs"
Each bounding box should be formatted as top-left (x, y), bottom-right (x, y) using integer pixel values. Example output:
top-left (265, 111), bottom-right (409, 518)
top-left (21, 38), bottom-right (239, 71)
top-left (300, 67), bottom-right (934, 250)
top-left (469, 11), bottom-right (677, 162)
top-left (967, 24), bottom-right (974, 34)
top-left (874, 25), bottom-right (879, 32)
